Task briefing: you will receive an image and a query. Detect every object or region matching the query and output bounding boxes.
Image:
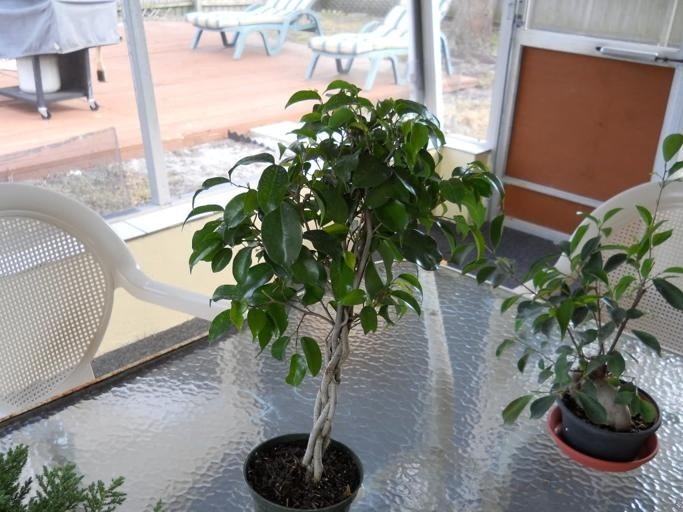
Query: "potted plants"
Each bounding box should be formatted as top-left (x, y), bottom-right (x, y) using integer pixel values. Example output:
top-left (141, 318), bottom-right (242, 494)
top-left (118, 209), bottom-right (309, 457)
top-left (456, 131), bottom-right (682, 474)
top-left (176, 78), bottom-right (518, 512)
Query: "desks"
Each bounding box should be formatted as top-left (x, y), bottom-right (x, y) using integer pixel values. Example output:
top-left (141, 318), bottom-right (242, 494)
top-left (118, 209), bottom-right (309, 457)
top-left (0, 252), bottom-right (683, 511)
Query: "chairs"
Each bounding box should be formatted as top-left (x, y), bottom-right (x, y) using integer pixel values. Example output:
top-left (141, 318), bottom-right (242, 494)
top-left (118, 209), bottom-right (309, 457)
top-left (304, 1), bottom-right (457, 97)
top-left (506, 177), bottom-right (682, 356)
top-left (184, 1), bottom-right (329, 62)
top-left (1, 178), bottom-right (246, 429)
